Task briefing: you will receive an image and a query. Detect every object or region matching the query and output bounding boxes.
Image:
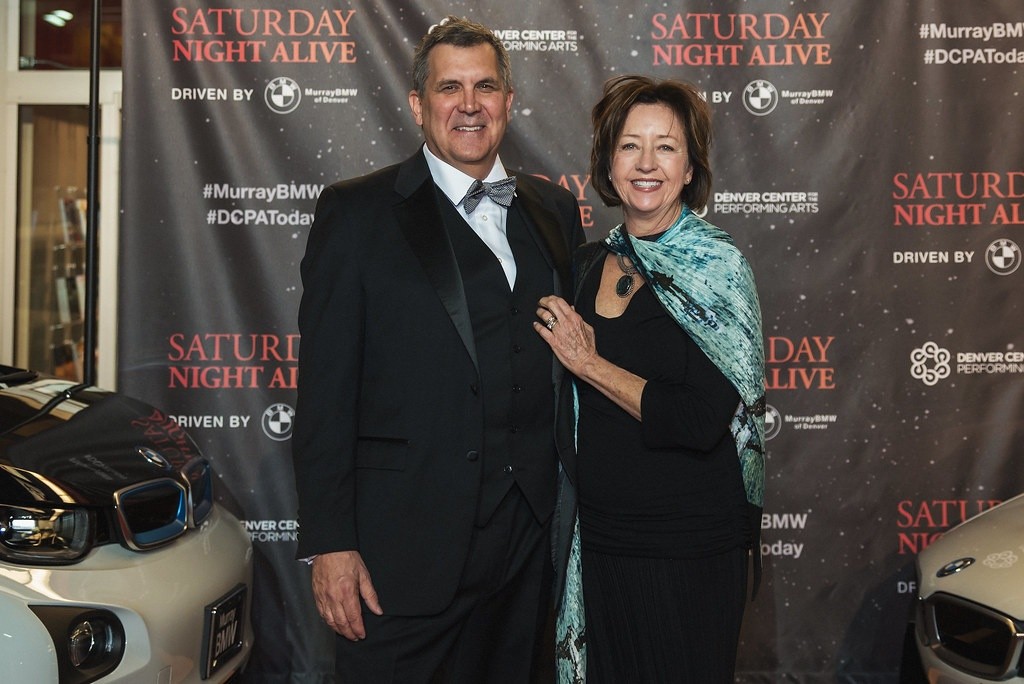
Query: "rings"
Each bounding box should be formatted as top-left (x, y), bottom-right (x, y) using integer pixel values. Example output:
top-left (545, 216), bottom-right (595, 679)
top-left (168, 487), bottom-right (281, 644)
top-left (546, 316), bottom-right (557, 328)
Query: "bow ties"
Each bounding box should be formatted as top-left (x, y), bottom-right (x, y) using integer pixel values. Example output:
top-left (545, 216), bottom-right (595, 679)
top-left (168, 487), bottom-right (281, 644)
top-left (464, 175), bottom-right (517, 214)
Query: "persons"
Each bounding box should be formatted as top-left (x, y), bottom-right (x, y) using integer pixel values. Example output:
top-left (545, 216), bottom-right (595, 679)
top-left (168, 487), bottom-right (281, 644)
top-left (295, 19), bottom-right (586, 684)
top-left (534, 75), bottom-right (766, 683)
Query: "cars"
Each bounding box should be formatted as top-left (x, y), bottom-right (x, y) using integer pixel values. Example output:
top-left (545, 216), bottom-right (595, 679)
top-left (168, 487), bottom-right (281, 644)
top-left (1, 364), bottom-right (257, 683)
top-left (914, 492), bottom-right (1024, 684)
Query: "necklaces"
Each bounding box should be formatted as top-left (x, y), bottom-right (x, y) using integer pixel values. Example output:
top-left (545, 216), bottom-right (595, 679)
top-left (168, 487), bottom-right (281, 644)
top-left (616, 254), bottom-right (637, 298)
